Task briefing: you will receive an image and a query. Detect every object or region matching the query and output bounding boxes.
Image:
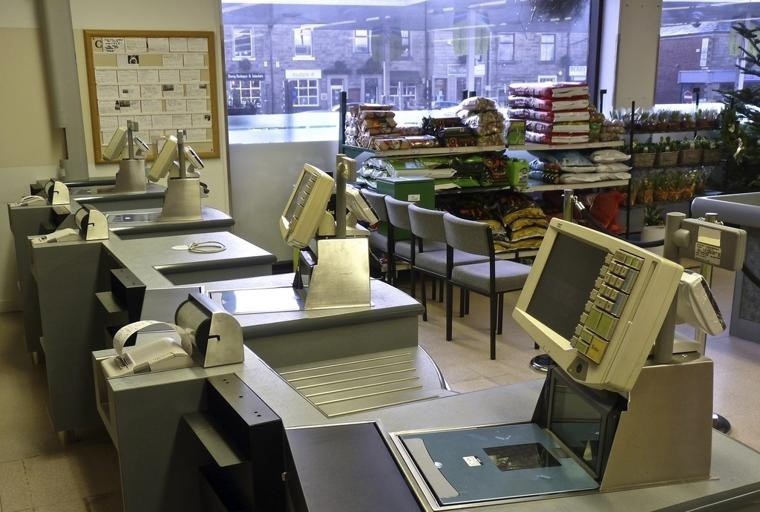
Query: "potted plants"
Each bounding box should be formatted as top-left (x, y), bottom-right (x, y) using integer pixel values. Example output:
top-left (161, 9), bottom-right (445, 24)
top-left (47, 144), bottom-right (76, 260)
top-left (609, 107), bottom-right (722, 243)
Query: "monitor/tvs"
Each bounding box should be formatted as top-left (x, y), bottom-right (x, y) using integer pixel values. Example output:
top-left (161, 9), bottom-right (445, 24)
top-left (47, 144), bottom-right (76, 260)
top-left (278, 162), bottom-right (336, 250)
top-left (510, 215), bottom-right (685, 394)
top-left (146, 134), bottom-right (178, 183)
top-left (103, 127), bottom-right (128, 161)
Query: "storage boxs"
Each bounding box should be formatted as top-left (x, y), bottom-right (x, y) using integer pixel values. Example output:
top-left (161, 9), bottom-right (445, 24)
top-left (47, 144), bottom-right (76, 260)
top-left (377, 176), bottom-right (435, 241)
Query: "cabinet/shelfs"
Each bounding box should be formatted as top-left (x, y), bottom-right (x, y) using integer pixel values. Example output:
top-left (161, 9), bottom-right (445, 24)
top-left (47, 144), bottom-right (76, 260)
top-left (600, 88), bottom-right (729, 248)
top-left (339, 91), bottom-right (635, 285)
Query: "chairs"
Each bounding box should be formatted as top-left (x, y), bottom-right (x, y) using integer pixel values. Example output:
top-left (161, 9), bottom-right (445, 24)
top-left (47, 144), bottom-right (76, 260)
top-left (361, 188), bottom-right (540, 359)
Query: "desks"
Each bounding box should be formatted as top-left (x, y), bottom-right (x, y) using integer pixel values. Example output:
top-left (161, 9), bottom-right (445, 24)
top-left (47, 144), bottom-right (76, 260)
top-left (691, 192), bottom-right (760, 343)
